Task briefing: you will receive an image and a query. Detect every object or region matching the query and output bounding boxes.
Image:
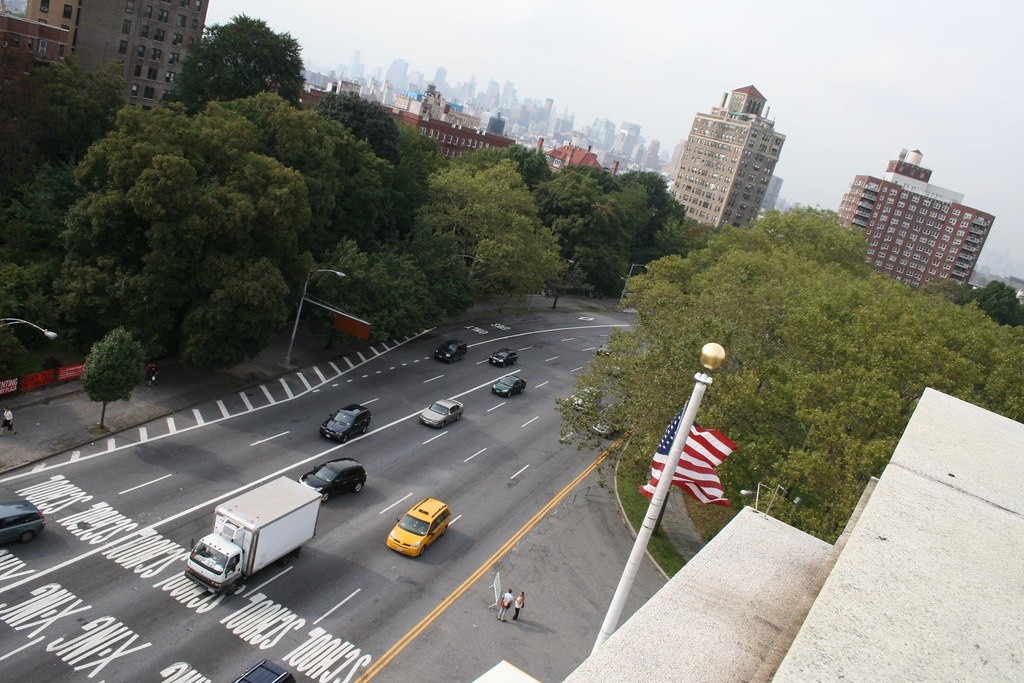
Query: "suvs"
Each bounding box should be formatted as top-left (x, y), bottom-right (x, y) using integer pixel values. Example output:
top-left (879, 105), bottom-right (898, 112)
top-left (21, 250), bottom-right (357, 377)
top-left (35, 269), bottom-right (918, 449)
top-left (231, 658), bottom-right (297, 683)
top-left (320, 403), bottom-right (372, 444)
top-left (299, 457), bottom-right (367, 503)
top-left (434, 338), bottom-right (467, 364)
top-left (386, 496), bottom-right (452, 557)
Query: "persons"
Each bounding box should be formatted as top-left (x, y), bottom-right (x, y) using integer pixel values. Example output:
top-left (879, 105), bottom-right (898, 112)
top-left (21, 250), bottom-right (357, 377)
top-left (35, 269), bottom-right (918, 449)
top-left (0, 406), bottom-right (17, 436)
top-left (144, 362), bottom-right (160, 388)
top-left (497, 588), bottom-right (514, 622)
top-left (513, 592), bottom-right (525, 621)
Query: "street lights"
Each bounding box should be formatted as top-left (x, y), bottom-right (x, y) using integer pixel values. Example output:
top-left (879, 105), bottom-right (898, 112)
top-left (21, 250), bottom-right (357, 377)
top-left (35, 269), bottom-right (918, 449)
top-left (283, 267), bottom-right (346, 366)
top-left (620, 262), bottom-right (649, 303)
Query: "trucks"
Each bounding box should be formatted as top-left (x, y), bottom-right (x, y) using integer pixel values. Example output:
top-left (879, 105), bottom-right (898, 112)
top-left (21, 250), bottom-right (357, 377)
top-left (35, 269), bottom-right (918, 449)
top-left (185, 474), bottom-right (324, 596)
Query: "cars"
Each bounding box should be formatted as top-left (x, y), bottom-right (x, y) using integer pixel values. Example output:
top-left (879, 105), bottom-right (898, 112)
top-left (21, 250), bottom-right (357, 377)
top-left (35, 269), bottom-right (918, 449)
top-left (0, 481), bottom-right (46, 546)
top-left (492, 375), bottom-right (528, 399)
top-left (419, 399), bottom-right (464, 429)
top-left (572, 387), bottom-right (602, 413)
top-left (488, 348), bottom-right (519, 368)
top-left (597, 343), bottom-right (614, 357)
top-left (592, 417), bottom-right (621, 438)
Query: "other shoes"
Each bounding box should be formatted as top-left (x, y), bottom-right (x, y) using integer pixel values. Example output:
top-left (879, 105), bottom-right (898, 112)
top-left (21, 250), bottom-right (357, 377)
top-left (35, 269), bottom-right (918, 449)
top-left (501, 619), bottom-right (506, 622)
top-left (498, 618), bottom-right (501, 620)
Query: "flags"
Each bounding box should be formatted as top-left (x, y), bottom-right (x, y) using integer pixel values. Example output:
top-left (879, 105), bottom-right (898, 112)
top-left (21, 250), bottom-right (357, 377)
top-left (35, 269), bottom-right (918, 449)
top-left (638, 401), bottom-right (737, 507)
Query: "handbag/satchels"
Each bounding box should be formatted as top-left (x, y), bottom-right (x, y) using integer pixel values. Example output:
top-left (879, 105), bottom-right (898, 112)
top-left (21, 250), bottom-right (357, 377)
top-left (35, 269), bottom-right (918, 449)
top-left (522, 602), bottom-right (524, 608)
